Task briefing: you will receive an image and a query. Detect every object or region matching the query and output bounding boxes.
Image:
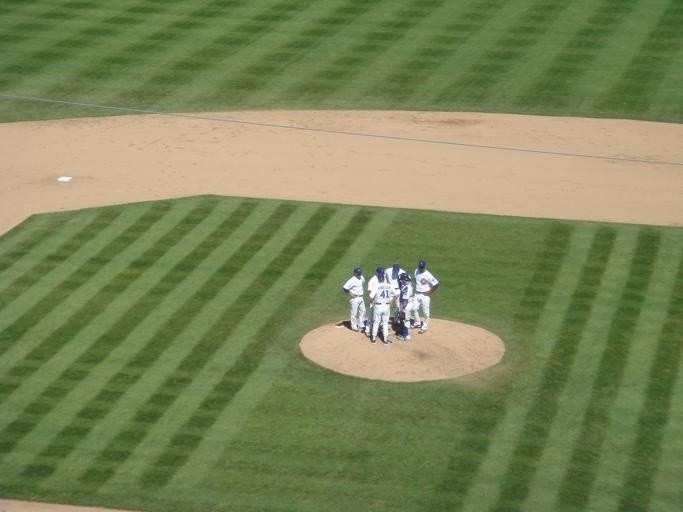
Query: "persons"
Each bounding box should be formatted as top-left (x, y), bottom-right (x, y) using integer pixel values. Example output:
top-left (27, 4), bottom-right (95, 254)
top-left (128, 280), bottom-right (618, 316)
top-left (411, 259), bottom-right (440, 334)
top-left (396, 272), bottom-right (413, 341)
top-left (364, 267), bottom-right (387, 338)
top-left (341, 267), bottom-right (366, 332)
top-left (384, 264), bottom-right (411, 329)
top-left (368, 274), bottom-right (397, 344)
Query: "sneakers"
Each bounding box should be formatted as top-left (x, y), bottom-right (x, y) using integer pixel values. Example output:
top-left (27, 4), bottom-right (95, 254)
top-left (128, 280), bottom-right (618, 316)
top-left (419, 329), bottom-right (426, 333)
top-left (371, 335), bottom-right (376, 344)
top-left (396, 328), bottom-right (411, 341)
top-left (358, 323), bottom-right (366, 328)
top-left (383, 337), bottom-right (391, 346)
top-left (365, 332), bottom-right (371, 339)
top-left (411, 321), bottom-right (421, 328)
top-left (353, 327), bottom-right (359, 332)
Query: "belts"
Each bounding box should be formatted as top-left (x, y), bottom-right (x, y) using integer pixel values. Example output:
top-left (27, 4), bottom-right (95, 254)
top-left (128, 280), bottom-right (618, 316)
top-left (355, 295), bottom-right (363, 297)
top-left (415, 291), bottom-right (424, 294)
top-left (395, 288), bottom-right (400, 289)
top-left (375, 303), bottom-right (389, 305)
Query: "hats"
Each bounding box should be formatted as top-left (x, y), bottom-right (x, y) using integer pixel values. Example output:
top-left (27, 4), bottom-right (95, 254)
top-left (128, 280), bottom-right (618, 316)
top-left (393, 264), bottom-right (400, 271)
top-left (399, 273), bottom-right (411, 281)
top-left (378, 273), bottom-right (385, 281)
top-left (418, 260), bottom-right (426, 268)
top-left (376, 267), bottom-right (385, 274)
top-left (353, 267), bottom-right (364, 274)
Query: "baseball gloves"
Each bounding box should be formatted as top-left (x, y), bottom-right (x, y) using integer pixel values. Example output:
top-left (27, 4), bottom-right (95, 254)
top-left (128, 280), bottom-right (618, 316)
top-left (397, 311), bottom-right (406, 320)
